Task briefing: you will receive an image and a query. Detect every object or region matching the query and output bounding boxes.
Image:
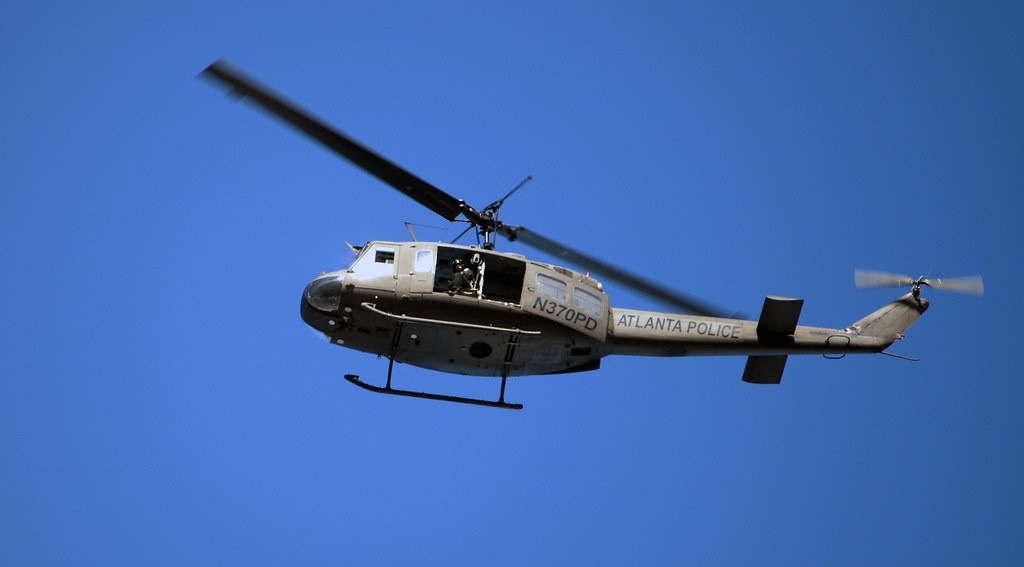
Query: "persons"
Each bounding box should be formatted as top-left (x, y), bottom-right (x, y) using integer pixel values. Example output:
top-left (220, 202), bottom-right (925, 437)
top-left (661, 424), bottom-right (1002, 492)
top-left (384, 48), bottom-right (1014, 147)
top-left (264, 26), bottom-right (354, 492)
top-left (447, 259), bottom-right (472, 295)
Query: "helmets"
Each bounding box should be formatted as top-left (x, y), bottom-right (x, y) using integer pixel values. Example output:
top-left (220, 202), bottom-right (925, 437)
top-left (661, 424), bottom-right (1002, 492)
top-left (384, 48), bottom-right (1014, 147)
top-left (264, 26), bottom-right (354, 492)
top-left (452, 259), bottom-right (466, 266)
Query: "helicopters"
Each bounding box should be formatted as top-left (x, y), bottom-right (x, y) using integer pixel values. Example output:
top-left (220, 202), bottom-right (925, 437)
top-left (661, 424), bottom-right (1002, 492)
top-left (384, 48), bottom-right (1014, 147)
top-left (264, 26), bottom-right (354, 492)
top-left (198, 59), bottom-right (1010, 412)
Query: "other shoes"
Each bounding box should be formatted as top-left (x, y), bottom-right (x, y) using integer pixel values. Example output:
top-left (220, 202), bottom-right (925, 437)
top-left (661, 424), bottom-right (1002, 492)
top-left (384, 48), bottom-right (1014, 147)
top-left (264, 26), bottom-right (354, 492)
top-left (447, 290), bottom-right (455, 296)
